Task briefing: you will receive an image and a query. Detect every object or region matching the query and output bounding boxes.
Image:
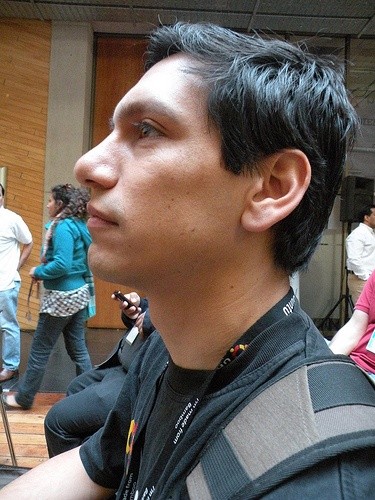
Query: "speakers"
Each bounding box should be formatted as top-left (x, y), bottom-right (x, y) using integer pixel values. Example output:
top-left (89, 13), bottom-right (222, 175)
top-left (340, 176), bottom-right (375, 224)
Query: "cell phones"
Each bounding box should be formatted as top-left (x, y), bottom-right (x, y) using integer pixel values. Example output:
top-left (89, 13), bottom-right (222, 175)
top-left (114, 291), bottom-right (137, 311)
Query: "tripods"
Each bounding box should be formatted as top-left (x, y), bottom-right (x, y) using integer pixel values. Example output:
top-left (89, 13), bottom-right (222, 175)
top-left (318, 222), bottom-right (355, 334)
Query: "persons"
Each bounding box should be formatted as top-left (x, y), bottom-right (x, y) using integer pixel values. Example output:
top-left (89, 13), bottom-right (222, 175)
top-left (345, 204), bottom-right (375, 306)
top-left (0, 184), bottom-right (34, 382)
top-left (44, 292), bottom-right (156, 459)
top-left (0, 22), bottom-right (375, 500)
top-left (3, 184), bottom-right (96, 409)
top-left (324, 266), bottom-right (375, 382)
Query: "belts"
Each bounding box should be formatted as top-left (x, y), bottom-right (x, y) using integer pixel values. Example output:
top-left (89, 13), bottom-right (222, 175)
top-left (348, 270), bottom-right (355, 273)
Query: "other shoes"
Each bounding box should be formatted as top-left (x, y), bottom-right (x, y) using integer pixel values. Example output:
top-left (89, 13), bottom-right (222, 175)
top-left (0, 394), bottom-right (30, 409)
top-left (0, 368), bottom-right (19, 383)
